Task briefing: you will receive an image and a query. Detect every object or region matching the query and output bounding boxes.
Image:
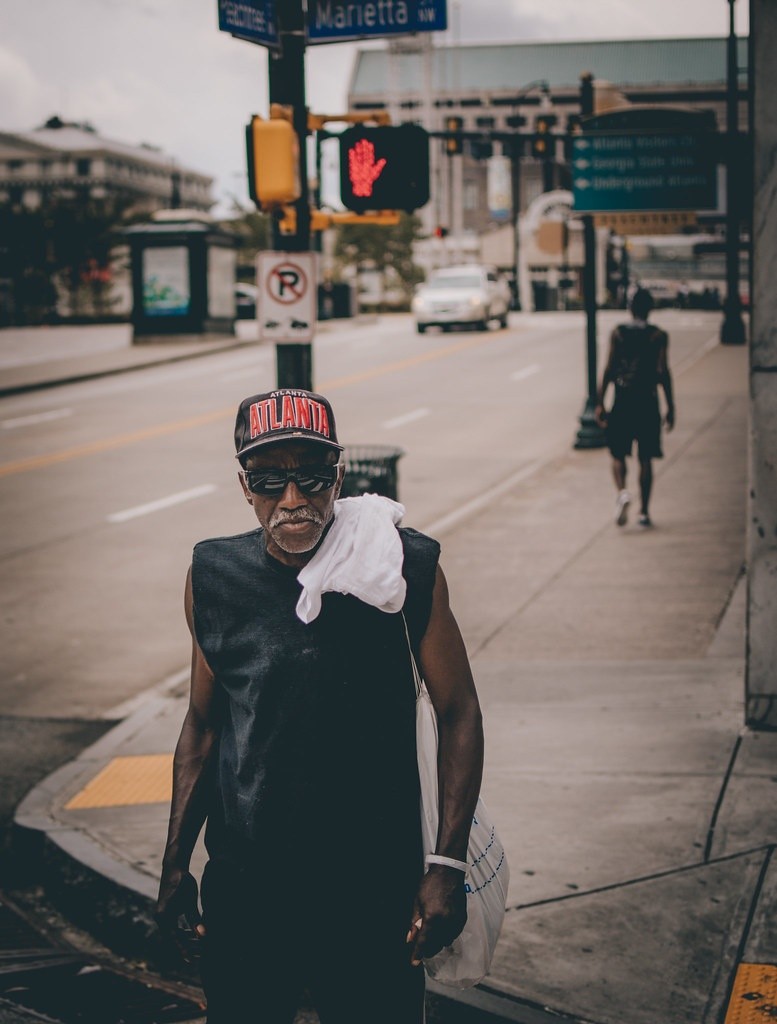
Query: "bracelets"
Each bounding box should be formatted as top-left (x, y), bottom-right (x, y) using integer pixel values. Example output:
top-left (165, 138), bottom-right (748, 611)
top-left (423, 853), bottom-right (473, 878)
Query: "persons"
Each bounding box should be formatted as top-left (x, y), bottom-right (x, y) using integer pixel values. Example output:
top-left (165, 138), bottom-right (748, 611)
top-left (592, 287), bottom-right (676, 526)
top-left (677, 279), bottom-right (691, 308)
top-left (146, 389), bottom-right (485, 1024)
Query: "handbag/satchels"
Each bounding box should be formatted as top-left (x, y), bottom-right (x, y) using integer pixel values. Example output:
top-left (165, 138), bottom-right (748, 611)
top-left (401, 614), bottom-right (508, 991)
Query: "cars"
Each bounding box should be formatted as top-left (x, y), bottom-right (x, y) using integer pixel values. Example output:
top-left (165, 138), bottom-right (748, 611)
top-left (411, 264), bottom-right (512, 332)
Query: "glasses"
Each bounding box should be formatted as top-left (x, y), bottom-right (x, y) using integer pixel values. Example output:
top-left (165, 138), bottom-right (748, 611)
top-left (244, 464), bottom-right (338, 497)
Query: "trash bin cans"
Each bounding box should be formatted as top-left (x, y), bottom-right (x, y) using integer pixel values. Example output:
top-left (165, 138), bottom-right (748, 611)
top-left (337, 443), bottom-right (406, 504)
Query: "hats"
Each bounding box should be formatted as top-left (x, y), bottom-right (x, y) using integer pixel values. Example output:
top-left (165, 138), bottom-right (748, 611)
top-left (234, 387), bottom-right (347, 455)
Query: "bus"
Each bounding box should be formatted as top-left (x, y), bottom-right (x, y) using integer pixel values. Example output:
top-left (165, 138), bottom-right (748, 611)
top-left (629, 226), bottom-right (754, 314)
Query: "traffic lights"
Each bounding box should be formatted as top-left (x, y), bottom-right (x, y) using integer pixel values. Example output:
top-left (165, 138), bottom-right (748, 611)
top-left (443, 115), bottom-right (463, 157)
top-left (340, 121), bottom-right (429, 216)
top-left (531, 115), bottom-right (553, 164)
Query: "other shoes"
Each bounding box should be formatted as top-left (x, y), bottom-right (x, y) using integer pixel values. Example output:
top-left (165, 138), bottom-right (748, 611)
top-left (616, 491), bottom-right (632, 528)
